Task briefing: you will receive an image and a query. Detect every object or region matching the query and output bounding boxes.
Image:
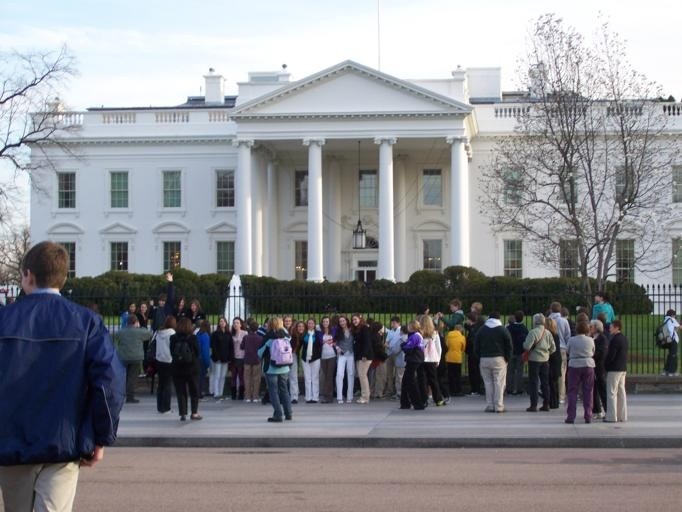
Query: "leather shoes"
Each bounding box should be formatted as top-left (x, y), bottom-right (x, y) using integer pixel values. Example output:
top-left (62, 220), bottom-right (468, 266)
top-left (527, 407), bottom-right (537, 411)
top-left (180, 416), bottom-right (186, 421)
top-left (126, 399), bottom-right (139, 403)
top-left (285, 416), bottom-right (292, 420)
top-left (540, 407), bottom-right (549, 411)
top-left (268, 418), bottom-right (283, 423)
top-left (190, 415), bottom-right (202, 420)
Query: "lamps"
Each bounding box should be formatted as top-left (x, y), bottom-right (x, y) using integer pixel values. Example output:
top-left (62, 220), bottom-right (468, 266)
top-left (352, 140), bottom-right (367, 250)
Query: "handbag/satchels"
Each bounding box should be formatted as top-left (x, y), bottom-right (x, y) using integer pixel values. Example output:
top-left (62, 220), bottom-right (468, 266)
top-left (522, 350), bottom-right (528, 363)
top-left (146, 331), bottom-right (158, 362)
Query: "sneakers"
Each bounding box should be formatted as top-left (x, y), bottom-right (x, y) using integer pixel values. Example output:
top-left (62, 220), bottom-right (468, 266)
top-left (485, 406), bottom-right (495, 412)
top-left (593, 413), bottom-right (602, 419)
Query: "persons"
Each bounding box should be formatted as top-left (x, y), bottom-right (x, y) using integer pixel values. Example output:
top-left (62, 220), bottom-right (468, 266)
top-left (379, 291), bottom-right (629, 425)
top-left (1, 240), bottom-right (124, 512)
top-left (660, 309), bottom-right (682, 378)
top-left (113, 269), bottom-right (211, 421)
top-left (256, 316), bottom-right (293, 421)
top-left (209, 314), bottom-right (394, 404)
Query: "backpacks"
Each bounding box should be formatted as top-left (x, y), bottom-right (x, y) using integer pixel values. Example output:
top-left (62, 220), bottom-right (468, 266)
top-left (270, 338), bottom-right (293, 367)
top-left (173, 334), bottom-right (193, 365)
top-left (654, 318), bottom-right (674, 349)
